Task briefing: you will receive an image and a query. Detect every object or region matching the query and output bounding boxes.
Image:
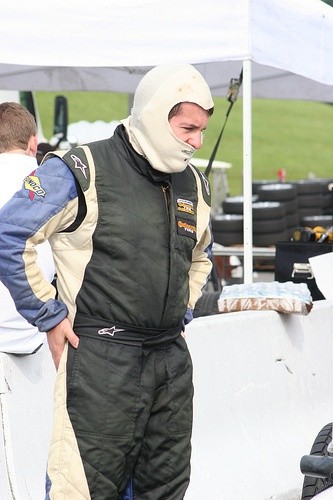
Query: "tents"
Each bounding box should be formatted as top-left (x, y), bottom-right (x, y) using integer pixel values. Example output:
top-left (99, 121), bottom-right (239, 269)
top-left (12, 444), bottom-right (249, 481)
top-left (0, 0), bottom-right (333, 313)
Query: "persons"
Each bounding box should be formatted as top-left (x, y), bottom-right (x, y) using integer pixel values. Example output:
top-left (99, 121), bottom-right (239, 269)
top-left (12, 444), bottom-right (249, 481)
top-left (0, 101), bottom-right (65, 499)
top-left (0, 62), bottom-right (216, 500)
